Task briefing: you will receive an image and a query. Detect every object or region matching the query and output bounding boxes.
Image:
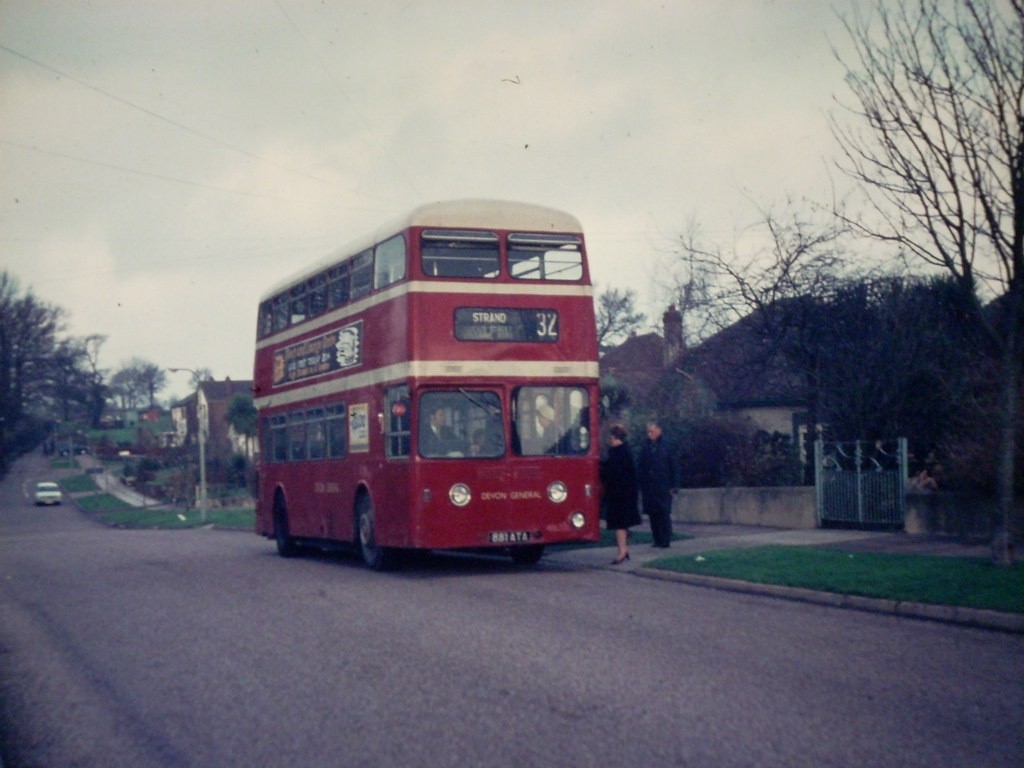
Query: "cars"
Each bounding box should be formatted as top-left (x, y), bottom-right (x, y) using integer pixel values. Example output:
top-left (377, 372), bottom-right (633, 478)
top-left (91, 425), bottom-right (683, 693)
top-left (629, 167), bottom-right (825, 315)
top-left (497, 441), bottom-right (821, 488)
top-left (34, 482), bottom-right (63, 506)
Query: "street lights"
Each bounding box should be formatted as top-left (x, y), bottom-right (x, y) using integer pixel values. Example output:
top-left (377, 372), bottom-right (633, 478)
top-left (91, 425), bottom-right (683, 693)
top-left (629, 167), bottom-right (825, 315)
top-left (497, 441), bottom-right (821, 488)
top-left (167, 367), bottom-right (209, 524)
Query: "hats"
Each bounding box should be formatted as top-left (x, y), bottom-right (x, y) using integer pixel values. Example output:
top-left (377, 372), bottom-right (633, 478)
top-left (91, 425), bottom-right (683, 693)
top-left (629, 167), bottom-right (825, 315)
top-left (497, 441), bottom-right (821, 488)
top-left (535, 403), bottom-right (554, 421)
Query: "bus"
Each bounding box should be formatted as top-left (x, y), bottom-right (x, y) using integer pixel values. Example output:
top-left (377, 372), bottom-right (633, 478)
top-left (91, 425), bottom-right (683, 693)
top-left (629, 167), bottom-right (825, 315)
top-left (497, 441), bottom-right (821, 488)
top-left (254, 198), bottom-right (602, 573)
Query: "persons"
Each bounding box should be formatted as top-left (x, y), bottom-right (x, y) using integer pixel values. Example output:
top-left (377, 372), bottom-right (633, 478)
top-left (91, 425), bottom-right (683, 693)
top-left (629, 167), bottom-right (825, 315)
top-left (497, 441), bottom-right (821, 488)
top-left (598, 418), bottom-right (678, 564)
top-left (470, 398), bottom-right (523, 458)
top-left (417, 404), bottom-right (470, 457)
top-left (534, 405), bottom-right (561, 456)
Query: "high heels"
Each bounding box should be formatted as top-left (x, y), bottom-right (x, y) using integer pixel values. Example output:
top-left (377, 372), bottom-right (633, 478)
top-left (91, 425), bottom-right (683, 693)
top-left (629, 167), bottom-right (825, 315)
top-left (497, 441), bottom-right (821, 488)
top-left (610, 552), bottom-right (631, 565)
